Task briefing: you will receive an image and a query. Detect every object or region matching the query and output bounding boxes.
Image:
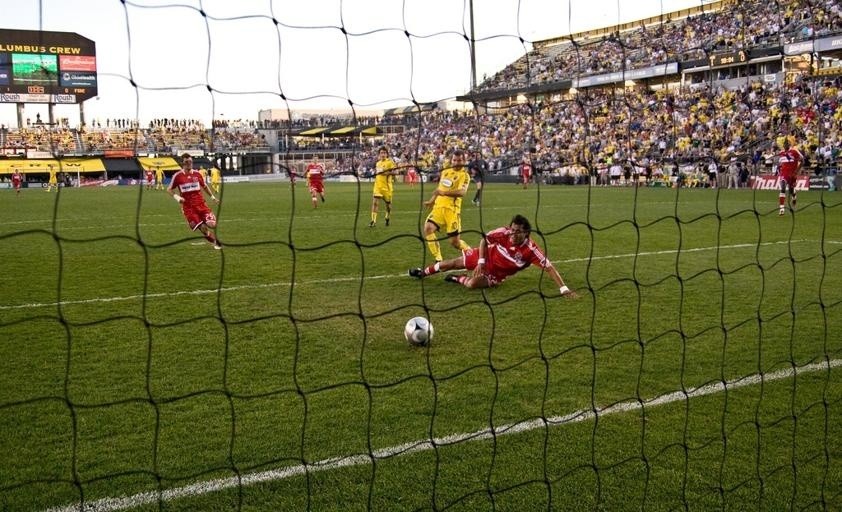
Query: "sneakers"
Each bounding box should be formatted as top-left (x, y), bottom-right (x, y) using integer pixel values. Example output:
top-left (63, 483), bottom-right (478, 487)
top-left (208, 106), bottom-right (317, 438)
top-left (385, 216), bottom-right (390, 226)
top-left (409, 268), bottom-right (421, 277)
top-left (368, 222), bottom-right (376, 227)
top-left (444, 274), bottom-right (458, 283)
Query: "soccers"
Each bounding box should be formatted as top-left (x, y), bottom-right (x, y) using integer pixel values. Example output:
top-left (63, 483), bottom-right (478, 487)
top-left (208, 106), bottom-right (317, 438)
top-left (404, 317), bottom-right (434, 345)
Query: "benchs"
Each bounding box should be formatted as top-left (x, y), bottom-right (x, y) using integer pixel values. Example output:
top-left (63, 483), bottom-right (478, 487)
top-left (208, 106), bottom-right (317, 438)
top-left (757, 161), bottom-right (842, 177)
top-left (0, 131), bottom-right (267, 153)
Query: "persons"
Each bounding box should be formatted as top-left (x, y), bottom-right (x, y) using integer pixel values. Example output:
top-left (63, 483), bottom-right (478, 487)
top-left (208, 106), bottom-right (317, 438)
top-left (468, 151), bottom-right (491, 206)
top-left (278, 1), bottom-right (842, 191)
top-left (1, 112), bottom-right (278, 192)
top-left (407, 214), bottom-right (579, 299)
top-left (167, 153), bottom-right (222, 250)
top-left (777, 139), bottom-right (805, 217)
top-left (423, 150), bottom-right (472, 262)
top-left (368, 146), bottom-right (396, 226)
top-left (303, 154), bottom-right (325, 208)
